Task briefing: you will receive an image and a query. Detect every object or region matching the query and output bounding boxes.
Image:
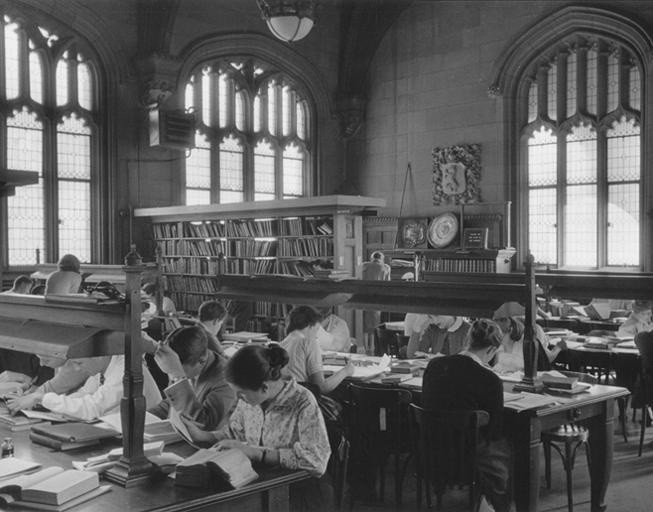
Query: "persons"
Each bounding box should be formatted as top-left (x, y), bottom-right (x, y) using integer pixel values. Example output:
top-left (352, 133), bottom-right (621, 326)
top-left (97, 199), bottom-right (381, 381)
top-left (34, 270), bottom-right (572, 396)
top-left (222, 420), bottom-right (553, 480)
top-left (194, 298), bottom-right (231, 359)
top-left (535, 298), bottom-right (567, 363)
top-left (44, 253), bottom-right (84, 296)
top-left (140, 282), bottom-right (183, 333)
top-left (144, 324), bottom-right (239, 432)
top-left (178, 342), bottom-right (332, 479)
top-left (421, 317), bottom-right (505, 442)
top-left (1, 274), bottom-right (33, 296)
top-left (617, 299), bottom-right (653, 338)
top-left (313, 307), bottom-right (352, 355)
top-left (6, 354), bottom-right (163, 422)
top-left (34, 352), bottom-right (91, 395)
top-left (406, 313), bottom-right (472, 359)
top-left (360, 250), bottom-right (392, 356)
top-left (278, 304), bottom-right (355, 422)
top-left (492, 317), bottom-right (525, 376)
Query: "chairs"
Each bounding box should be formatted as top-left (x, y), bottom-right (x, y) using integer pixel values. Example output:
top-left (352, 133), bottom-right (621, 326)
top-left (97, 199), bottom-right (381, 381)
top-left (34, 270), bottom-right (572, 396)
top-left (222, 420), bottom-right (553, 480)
top-left (339, 383), bottom-right (414, 512)
top-left (405, 399), bottom-right (502, 510)
top-left (632, 330), bottom-right (653, 456)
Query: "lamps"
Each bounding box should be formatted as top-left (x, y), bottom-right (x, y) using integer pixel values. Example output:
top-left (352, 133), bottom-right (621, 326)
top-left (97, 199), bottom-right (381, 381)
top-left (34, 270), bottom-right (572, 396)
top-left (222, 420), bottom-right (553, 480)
top-left (260, 0), bottom-right (316, 42)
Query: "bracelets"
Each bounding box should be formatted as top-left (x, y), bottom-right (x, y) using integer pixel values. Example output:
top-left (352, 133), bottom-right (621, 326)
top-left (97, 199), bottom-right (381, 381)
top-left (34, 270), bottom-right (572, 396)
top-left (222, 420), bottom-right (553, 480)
top-left (260, 448), bottom-right (267, 463)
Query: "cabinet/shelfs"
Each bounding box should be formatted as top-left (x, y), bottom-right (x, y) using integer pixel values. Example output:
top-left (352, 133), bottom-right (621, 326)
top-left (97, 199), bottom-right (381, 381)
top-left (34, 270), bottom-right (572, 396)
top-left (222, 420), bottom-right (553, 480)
top-left (31, 264), bottom-right (161, 338)
top-left (136, 195), bottom-right (387, 350)
top-left (538, 295), bottom-right (626, 333)
top-left (0, 247), bottom-right (314, 512)
top-left (390, 319), bottom-right (653, 439)
top-left (214, 256), bottom-right (628, 512)
top-left (364, 251), bottom-right (516, 284)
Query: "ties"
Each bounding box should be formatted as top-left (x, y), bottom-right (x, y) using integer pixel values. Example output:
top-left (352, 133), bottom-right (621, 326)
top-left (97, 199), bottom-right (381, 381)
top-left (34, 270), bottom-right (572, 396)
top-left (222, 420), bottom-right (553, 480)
top-left (100, 374), bottom-right (105, 385)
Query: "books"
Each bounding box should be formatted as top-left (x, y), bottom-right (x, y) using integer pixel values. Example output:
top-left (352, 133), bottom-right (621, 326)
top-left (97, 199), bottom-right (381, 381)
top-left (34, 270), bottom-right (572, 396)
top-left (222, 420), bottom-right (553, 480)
top-left (71, 440), bottom-right (185, 473)
top-left (424, 257), bottom-right (497, 274)
top-left (151, 215), bottom-right (352, 282)
top-left (0, 415), bottom-right (53, 432)
top-left (0, 484), bottom-right (113, 512)
top-left (549, 298), bottom-right (628, 323)
top-left (505, 369), bottom-right (591, 413)
top-left (174, 447), bottom-right (260, 491)
top-left (92, 412), bottom-right (183, 444)
top-left (223, 330), bottom-right (271, 341)
top-left (244, 318), bottom-right (267, 333)
top-left (19, 408), bottom-right (100, 424)
top-left (0, 465), bottom-right (100, 506)
top-left (547, 327), bottom-right (637, 352)
top-left (160, 274), bottom-right (298, 315)
top-left (29, 422), bottom-right (122, 450)
top-left (0, 457), bottom-right (42, 482)
top-left (320, 350), bottom-right (446, 391)
